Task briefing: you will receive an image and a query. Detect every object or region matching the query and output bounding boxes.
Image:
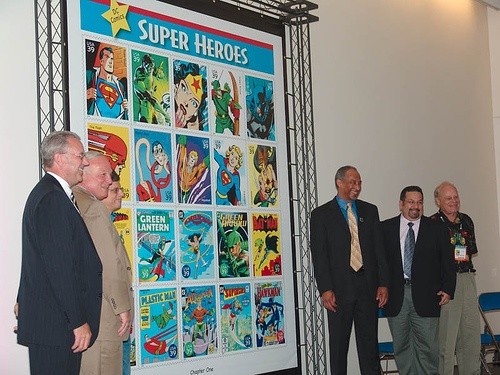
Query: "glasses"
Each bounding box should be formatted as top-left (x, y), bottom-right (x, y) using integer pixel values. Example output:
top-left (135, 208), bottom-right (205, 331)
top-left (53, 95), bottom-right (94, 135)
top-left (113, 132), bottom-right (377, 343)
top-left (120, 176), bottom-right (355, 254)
top-left (60, 152), bottom-right (85, 160)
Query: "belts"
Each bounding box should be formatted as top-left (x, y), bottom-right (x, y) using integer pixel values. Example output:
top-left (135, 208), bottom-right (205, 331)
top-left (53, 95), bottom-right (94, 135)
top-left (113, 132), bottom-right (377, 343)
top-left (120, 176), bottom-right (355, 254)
top-left (403, 278), bottom-right (411, 284)
top-left (456, 268), bottom-right (476, 273)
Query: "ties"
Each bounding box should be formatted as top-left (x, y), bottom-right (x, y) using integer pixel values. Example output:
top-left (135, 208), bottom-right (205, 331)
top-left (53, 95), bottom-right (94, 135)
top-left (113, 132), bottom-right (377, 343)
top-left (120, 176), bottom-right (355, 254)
top-left (347, 203), bottom-right (363, 273)
top-left (404, 222), bottom-right (415, 278)
top-left (71, 192), bottom-right (80, 213)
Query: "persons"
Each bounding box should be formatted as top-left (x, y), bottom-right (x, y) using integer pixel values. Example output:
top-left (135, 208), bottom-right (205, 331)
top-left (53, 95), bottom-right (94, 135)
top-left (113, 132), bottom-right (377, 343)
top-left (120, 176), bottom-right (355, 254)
top-left (307, 165), bottom-right (390, 375)
top-left (13, 133), bottom-right (102, 375)
top-left (101, 169), bottom-right (123, 214)
top-left (72, 151), bottom-right (133, 375)
top-left (426, 180), bottom-right (482, 375)
top-left (379, 185), bottom-right (457, 375)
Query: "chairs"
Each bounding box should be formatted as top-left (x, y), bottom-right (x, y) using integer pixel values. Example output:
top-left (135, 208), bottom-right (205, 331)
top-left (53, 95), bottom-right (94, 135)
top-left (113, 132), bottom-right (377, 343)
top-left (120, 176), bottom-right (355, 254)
top-left (377, 307), bottom-right (400, 375)
top-left (478, 292), bottom-right (500, 375)
